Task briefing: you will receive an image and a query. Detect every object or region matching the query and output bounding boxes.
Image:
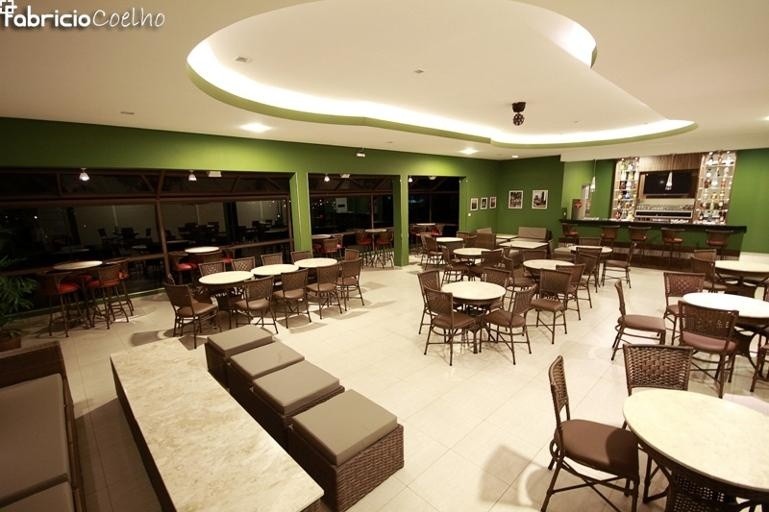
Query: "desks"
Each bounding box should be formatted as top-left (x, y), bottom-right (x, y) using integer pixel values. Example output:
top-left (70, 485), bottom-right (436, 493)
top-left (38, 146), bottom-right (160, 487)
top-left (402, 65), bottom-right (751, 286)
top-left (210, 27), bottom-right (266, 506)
top-left (110, 336), bottom-right (325, 511)
top-left (624, 258), bottom-right (769, 511)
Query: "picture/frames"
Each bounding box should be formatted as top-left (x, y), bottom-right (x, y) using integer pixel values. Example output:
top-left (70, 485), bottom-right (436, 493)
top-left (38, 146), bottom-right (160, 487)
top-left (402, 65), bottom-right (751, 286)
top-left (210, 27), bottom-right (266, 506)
top-left (531, 189), bottom-right (549, 209)
top-left (470, 196), bottom-right (496, 212)
top-left (508, 190), bottom-right (523, 210)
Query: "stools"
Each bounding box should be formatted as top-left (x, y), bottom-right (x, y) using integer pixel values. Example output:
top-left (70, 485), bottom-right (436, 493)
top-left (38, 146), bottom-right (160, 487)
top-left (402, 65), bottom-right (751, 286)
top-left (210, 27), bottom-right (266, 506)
top-left (202, 324), bottom-right (405, 511)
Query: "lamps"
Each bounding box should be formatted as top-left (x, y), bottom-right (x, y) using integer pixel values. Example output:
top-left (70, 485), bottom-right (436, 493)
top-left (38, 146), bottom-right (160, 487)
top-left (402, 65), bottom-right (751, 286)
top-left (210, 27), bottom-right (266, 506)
top-left (79, 168), bottom-right (89, 182)
top-left (511, 101), bottom-right (527, 126)
top-left (187, 170), bottom-right (197, 182)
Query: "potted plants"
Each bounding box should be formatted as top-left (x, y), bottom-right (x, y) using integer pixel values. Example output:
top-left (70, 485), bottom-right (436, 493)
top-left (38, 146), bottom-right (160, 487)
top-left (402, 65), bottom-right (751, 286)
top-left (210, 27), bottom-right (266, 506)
top-left (0, 256), bottom-right (39, 349)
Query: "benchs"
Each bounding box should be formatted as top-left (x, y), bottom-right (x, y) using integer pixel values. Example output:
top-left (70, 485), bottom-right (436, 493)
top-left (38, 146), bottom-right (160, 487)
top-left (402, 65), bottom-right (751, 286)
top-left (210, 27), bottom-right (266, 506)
top-left (0, 340), bottom-right (87, 512)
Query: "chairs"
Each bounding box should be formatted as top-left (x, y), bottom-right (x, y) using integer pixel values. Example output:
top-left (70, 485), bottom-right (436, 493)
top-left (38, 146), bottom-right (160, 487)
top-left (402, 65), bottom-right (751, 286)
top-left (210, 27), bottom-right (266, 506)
top-left (39, 256), bottom-right (136, 339)
top-left (163, 224), bottom-right (414, 350)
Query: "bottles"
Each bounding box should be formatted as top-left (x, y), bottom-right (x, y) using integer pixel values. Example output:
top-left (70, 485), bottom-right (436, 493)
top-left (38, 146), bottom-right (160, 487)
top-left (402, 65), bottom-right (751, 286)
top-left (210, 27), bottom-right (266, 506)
top-left (696, 149), bottom-right (735, 224)
top-left (615, 156), bottom-right (638, 220)
